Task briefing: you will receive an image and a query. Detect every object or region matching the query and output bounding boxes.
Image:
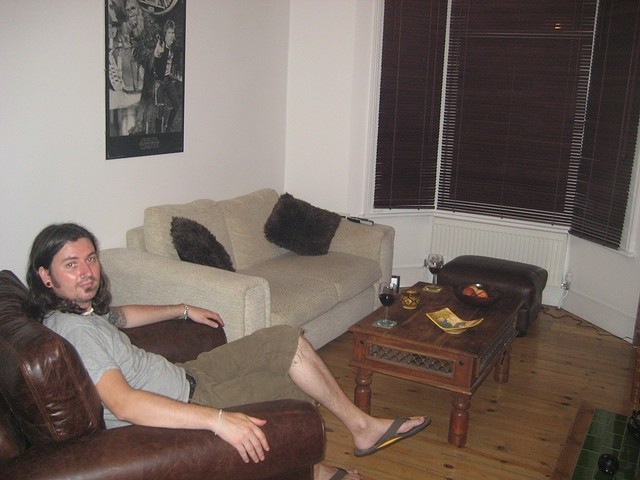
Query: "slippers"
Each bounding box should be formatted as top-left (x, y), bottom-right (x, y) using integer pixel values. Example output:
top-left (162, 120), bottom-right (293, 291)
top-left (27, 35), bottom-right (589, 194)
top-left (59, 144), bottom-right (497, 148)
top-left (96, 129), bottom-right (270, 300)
top-left (354, 415), bottom-right (432, 457)
top-left (328, 466), bottom-right (364, 480)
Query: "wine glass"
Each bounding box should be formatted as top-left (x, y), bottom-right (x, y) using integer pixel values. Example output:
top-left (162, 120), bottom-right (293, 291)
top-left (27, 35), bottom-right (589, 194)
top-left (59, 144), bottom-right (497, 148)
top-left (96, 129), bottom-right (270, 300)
top-left (374, 282), bottom-right (397, 327)
top-left (427, 254), bottom-right (444, 289)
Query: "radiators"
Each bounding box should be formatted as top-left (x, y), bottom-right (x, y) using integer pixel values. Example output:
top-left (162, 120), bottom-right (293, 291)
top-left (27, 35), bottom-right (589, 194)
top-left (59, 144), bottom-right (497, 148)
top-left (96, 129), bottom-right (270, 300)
top-left (430, 215), bottom-right (572, 309)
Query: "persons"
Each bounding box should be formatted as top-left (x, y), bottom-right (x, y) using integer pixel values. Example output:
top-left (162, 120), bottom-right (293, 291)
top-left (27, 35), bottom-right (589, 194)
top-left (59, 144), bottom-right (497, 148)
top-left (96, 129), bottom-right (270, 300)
top-left (24, 223), bottom-right (432, 480)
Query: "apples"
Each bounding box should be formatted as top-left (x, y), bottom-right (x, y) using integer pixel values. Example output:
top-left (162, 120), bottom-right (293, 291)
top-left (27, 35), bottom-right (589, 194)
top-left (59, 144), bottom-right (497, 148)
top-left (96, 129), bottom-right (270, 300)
top-left (464, 280), bottom-right (487, 298)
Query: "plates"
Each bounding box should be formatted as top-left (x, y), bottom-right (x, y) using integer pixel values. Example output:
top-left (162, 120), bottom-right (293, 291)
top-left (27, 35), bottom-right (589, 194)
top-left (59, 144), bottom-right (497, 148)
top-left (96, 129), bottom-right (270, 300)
top-left (450, 275), bottom-right (502, 306)
top-left (425, 308), bottom-right (484, 335)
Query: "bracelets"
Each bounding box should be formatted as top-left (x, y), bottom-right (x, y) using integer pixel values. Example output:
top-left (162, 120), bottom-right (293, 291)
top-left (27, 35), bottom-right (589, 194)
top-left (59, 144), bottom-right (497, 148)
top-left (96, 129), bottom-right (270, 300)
top-left (214, 409), bottom-right (223, 436)
top-left (180, 303), bottom-right (189, 320)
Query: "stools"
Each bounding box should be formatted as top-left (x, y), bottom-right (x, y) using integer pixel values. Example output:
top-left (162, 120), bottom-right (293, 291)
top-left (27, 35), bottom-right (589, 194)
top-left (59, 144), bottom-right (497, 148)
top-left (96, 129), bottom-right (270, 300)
top-left (437, 254), bottom-right (548, 338)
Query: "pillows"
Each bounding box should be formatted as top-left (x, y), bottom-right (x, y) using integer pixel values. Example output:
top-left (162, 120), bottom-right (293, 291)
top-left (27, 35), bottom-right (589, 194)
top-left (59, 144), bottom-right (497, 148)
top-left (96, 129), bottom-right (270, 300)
top-left (264, 192), bottom-right (340, 255)
top-left (169, 216), bottom-right (235, 271)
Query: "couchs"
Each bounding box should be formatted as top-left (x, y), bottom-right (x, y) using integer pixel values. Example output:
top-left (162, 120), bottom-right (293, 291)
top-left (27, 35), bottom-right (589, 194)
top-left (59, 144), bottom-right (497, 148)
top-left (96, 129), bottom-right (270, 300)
top-left (98, 189), bottom-right (396, 350)
top-left (0, 270), bottom-right (324, 480)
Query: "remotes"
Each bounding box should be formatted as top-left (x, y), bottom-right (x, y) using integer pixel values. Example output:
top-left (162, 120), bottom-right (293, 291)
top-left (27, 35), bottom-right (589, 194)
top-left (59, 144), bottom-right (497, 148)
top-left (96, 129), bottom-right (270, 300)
top-left (348, 217), bottom-right (374, 226)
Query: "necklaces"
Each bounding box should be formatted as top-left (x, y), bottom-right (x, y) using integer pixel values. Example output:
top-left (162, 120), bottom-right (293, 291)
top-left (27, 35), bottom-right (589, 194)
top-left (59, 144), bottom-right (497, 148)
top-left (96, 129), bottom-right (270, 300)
top-left (77, 308), bottom-right (96, 315)
